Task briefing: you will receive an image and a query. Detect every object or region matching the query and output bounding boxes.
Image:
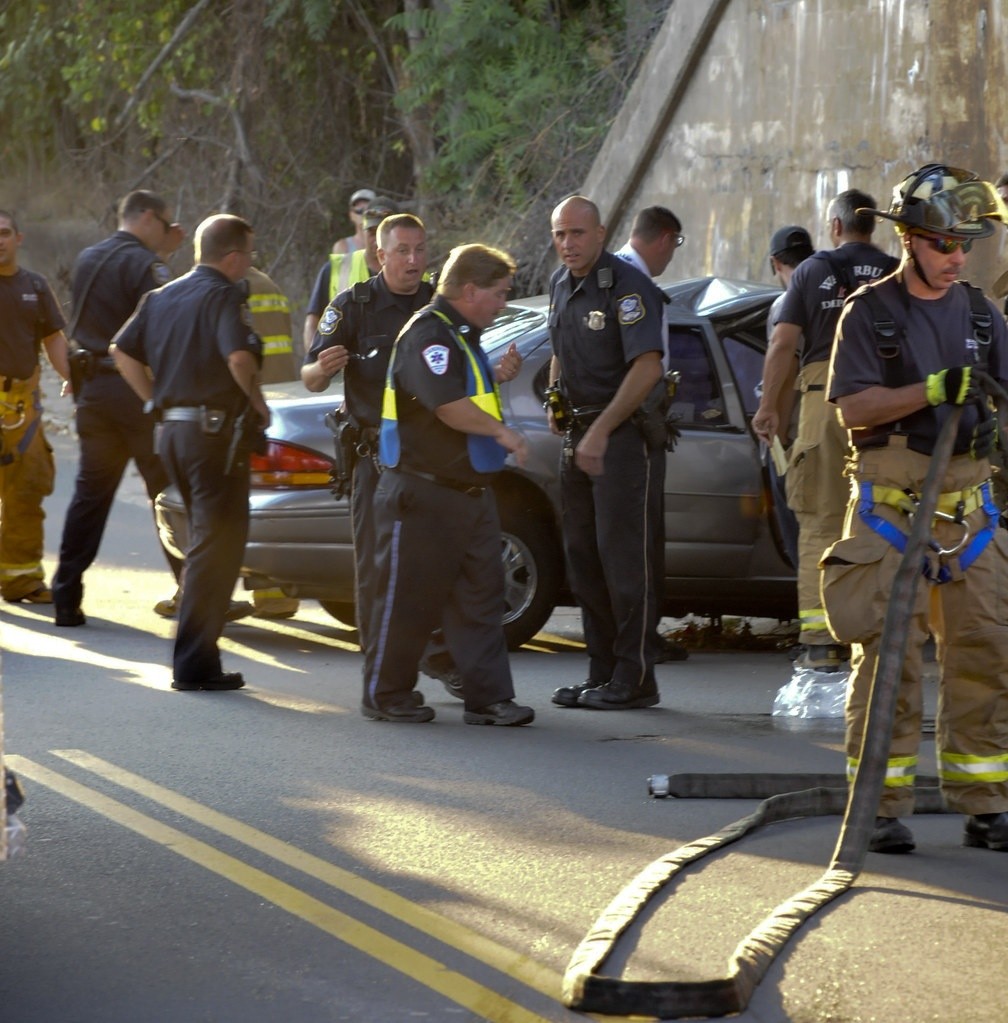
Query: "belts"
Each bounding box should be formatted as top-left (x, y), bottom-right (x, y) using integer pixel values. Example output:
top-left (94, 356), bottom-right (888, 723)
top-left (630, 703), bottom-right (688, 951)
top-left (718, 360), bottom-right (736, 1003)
top-left (856, 432), bottom-right (970, 456)
top-left (161, 406), bottom-right (200, 421)
top-left (396, 463), bottom-right (485, 496)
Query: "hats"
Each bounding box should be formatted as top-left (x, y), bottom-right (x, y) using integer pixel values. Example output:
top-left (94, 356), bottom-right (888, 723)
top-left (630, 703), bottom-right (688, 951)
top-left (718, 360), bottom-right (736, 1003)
top-left (770, 226), bottom-right (809, 255)
top-left (352, 190), bottom-right (376, 203)
top-left (362, 196), bottom-right (399, 229)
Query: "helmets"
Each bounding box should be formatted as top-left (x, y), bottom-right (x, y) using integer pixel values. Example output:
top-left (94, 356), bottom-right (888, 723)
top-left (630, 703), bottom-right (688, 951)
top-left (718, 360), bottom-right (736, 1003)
top-left (888, 167), bottom-right (1008, 239)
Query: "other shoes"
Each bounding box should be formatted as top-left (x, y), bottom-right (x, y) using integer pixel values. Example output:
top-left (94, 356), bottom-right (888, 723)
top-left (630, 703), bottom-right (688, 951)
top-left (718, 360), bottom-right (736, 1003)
top-left (56, 610), bottom-right (87, 626)
top-left (650, 635), bottom-right (688, 663)
top-left (792, 644), bottom-right (840, 672)
top-left (172, 672), bottom-right (245, 689)
top-left (4, 588), bottom-right (54, 603)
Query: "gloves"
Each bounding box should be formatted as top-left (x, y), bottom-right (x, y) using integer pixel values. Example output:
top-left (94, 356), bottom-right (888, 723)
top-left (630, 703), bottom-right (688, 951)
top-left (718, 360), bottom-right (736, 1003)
top-left (970, 403), bottom-right (997, 460)
top-left (927, 362), bottom-right (1008, 407)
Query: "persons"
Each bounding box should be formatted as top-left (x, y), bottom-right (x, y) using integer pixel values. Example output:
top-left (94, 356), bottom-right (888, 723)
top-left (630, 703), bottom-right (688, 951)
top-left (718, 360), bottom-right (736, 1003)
top-left (300, 191), bottom-right (540, 729)
top-left (752, 186), bottom-right (902, 672)
top-left (109, 213), bottom-right (275, 692)
top-left (235, 264), bottom-right (296, 383)
top-left (831, 161), bottom-right (1006, 856)
top-left (49, 188), bottom-right (185, 626)
top-left (0, 206), bottom-right (73, 606)
top-left (544, 195), bottom-right (691, 707)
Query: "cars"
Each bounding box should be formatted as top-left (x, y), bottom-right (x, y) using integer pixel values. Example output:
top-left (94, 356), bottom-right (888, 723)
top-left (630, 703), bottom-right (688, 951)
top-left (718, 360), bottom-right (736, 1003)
top-left (155, 275), bottom-right (799, 644)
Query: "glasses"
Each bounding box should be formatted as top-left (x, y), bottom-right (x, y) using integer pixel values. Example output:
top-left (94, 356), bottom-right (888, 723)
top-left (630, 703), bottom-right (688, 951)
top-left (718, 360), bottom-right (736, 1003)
top-left (155, 214), bottom-right (169, 232)
top-left (910, 233), bottom-right (971, 254)
top-left (674, 231), bottom-right (685, 245)
top-left (224, 250), bottom-right (259, 261)
top-left (354, 208), bottom-right (363, 214)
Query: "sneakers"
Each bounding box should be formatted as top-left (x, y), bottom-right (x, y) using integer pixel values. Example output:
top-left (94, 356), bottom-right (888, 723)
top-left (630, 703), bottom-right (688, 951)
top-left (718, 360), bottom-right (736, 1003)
top-left (360, 700), bottom-right (435, 722)
top-left (463, 699), bottom-right (534, 725)
top-left (869, 817), bottom-right (916, 851)
top-left (964, 813), bottom-right (1008, 849)
top-left (578, 679), bottom-right (660, 709)
top-left (551, 679), bottom-right (605, 704)
top-left (420, 664), bottom-right (466, 699)
